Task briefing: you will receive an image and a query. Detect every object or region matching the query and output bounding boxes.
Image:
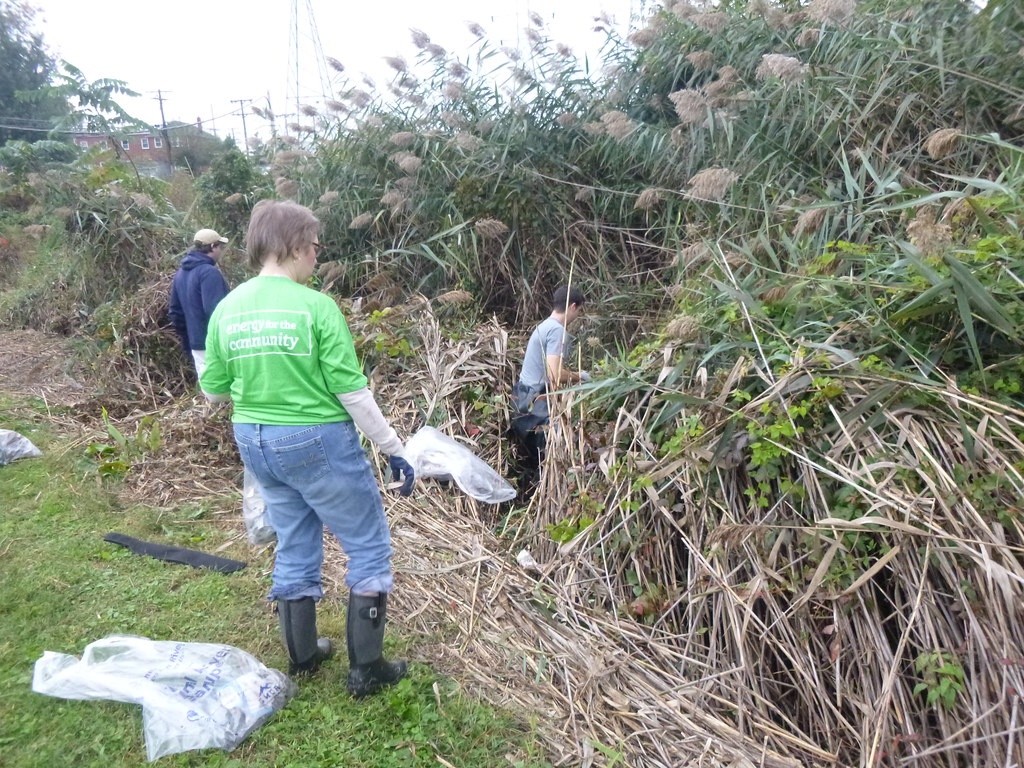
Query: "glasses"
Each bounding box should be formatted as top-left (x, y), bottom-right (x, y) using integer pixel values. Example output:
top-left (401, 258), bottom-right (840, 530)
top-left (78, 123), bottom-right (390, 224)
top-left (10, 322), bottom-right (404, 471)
top-left (302, 238), bottom-right (324, 258)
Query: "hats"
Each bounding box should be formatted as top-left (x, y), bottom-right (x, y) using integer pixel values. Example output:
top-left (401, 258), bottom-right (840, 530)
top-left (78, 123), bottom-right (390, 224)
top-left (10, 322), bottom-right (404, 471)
top-left (193, 229), bottom-right (229, 245)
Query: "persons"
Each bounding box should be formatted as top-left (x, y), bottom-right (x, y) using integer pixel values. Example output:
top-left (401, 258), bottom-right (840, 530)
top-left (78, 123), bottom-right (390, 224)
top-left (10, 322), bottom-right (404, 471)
top-left (515, 285), bottom-right (591, 506)
top-left (169, 229), bottom-right (230, 375)
top-left (199, 201), bottom-right (416, 697)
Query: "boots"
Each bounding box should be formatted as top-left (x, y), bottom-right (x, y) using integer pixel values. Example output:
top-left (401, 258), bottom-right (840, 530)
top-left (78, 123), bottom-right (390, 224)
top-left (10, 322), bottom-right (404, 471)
top-left (346, 591), bottom-right (407, 701)
top-left (276, 596), bottom-right (331, 680)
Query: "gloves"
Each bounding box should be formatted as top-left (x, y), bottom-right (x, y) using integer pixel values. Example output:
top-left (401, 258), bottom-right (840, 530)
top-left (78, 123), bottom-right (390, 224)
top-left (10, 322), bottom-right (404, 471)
top-left (578, 370), bottom-right (592, 381)
top-left (183, 344), bottom-right (193, 360)
top-left (389, 444), bottom-right (416, 497)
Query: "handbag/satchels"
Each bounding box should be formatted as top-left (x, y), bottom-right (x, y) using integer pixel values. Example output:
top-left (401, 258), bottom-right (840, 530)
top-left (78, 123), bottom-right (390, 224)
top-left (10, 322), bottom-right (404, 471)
top-left (242, 463), bottom-right (279, 546)
